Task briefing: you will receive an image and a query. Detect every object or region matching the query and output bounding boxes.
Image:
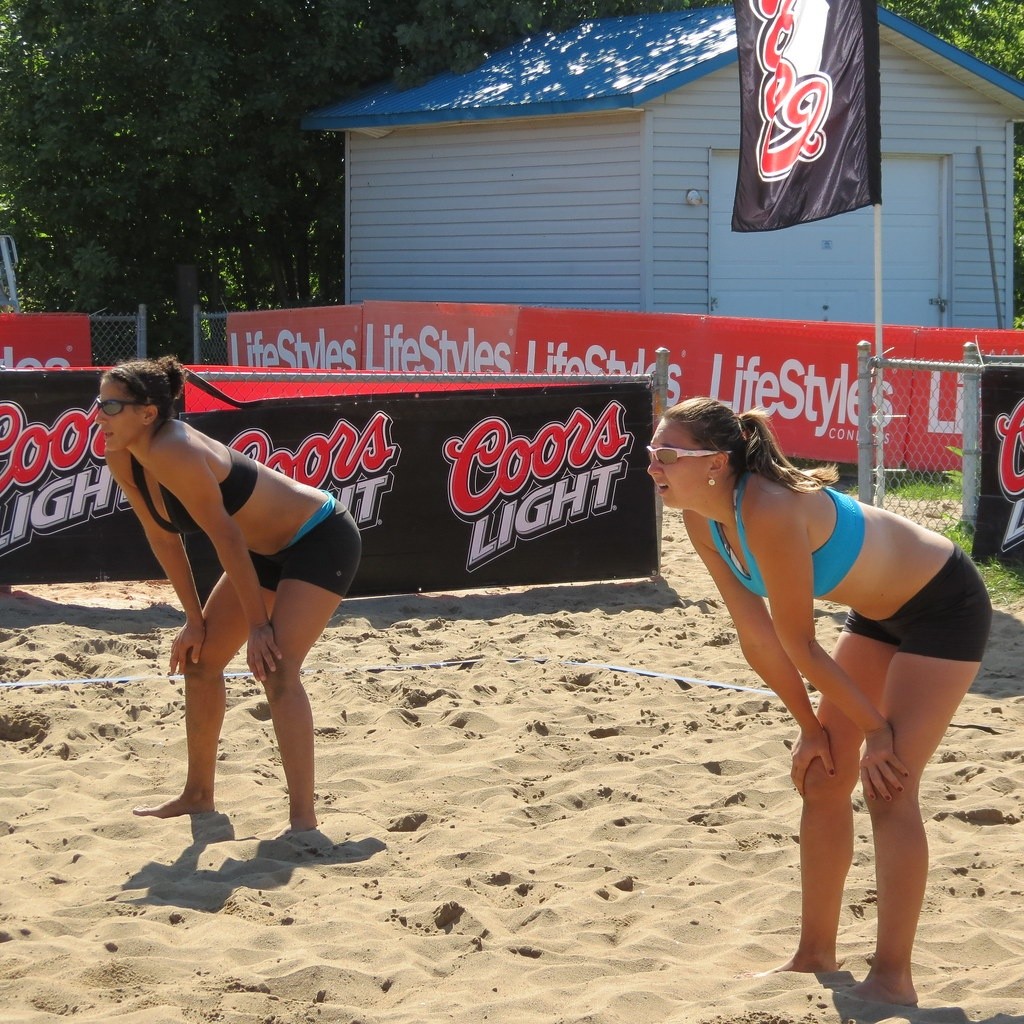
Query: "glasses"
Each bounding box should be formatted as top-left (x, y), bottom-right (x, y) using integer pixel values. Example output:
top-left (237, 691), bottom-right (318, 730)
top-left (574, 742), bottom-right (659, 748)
top-left (645, 445), bottom-right (733, 466)
top-left (95, 394), bottom-right (147, 416)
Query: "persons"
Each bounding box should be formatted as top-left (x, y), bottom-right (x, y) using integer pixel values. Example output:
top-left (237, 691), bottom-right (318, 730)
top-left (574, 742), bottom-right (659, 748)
top-left (97, 356), bottom-right (363, 845)
top-left (647, 397), bottom-right (992, 1008)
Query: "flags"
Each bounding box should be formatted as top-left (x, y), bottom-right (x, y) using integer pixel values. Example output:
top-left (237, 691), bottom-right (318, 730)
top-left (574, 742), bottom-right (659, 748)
top-left (731, 1), bottom-right (882, 232)
